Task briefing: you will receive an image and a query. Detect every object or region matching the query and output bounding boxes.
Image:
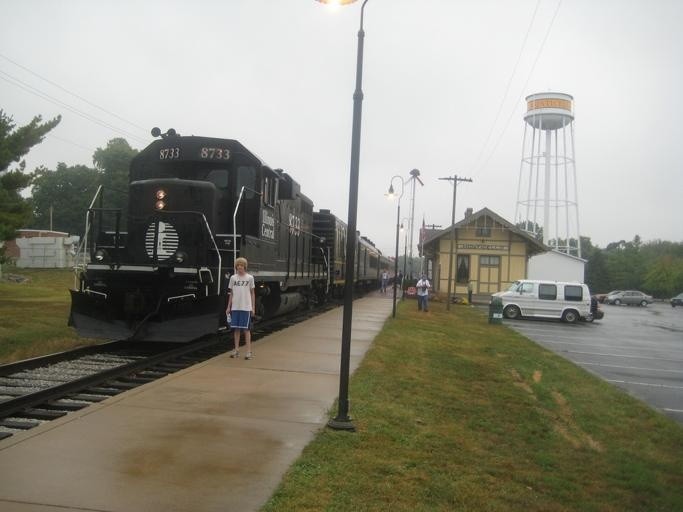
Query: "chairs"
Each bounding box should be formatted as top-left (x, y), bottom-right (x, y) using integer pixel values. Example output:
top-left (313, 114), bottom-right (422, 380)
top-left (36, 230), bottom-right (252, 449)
top-left (230, 349), bottom-right (241, 358)
top-left (245, 352), bottom-right (253, 360)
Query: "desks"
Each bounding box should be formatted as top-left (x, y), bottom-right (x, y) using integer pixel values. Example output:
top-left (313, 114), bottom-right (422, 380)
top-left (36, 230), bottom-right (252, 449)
top-left (399, 215), bottom-right (411, 280)
top-left (313, 1), bottom-right (368, 434)
top-left (387, 174), bottom-right (405, 319)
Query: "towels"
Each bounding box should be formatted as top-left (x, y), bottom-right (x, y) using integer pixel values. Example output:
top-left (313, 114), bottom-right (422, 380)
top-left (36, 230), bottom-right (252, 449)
top-left (407, 280), bottom-right (416, 297)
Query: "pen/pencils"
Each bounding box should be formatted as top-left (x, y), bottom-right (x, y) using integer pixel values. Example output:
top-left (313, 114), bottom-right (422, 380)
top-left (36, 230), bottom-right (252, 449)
top-left (488, 277), bottom-right (595, 324)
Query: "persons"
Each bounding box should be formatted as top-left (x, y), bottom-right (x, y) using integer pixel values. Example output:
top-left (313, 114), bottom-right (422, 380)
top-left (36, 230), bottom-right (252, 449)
top-left (224, 258), bottom-right (255, 360)
top-left (398, 270), bottom-right (402, 289)
top-left (415, 275), bottom-right (430, 312)
top-left (379, 268), bottom-right (388, 295)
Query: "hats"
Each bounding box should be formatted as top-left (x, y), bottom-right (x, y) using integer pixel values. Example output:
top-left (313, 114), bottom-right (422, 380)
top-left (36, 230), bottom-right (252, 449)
top-left (587, 295), bottom-right (604, 322)
top-left (668, 292), bottom-right (682, 308)
top-left (593, 289), bottom-right (654, 307)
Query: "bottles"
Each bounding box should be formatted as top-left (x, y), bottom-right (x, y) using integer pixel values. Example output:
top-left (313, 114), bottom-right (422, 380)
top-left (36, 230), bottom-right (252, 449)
top-left (422, 274), bottom-right (427, 280)
